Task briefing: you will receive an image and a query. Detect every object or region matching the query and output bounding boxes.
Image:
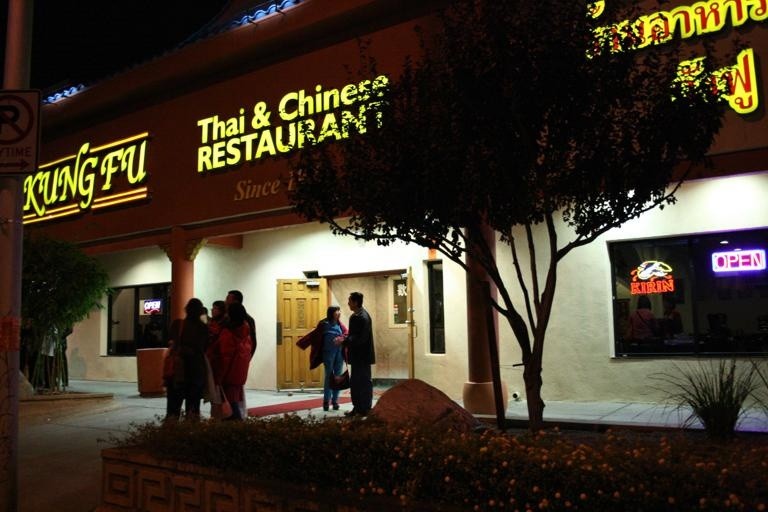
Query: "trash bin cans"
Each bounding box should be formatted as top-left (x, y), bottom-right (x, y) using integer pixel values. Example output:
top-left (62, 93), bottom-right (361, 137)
top-left (137, 348), bottom-right (167, 396)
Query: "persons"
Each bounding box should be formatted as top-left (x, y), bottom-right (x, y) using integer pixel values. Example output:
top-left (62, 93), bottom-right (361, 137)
top-left (663, 297), bottom-right (681, 324)
top-left (337, 292), bottom-right (375, 417)
top-left (211, 303), bottom-right (252, 421)
top-left (20, 317), bottom-right (73, 390)
top-left (163, 298), bottom-right (209, 428)
top-left (627, 295), bottom-right (659, 347)
top-left (296, 306), bottom-right (348, 410)
top-left (207, 300), bottom-right (226, 337)
top-left (226, 291), bottom-right (257, 356)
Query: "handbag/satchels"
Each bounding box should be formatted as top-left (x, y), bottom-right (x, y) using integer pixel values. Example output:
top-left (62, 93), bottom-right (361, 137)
top-left (163, 354), bottom-right (181, 377)
top-left (328, 369), bottom-right (350, 390)
top-left (211, 402), bottom-right (233, 419)
